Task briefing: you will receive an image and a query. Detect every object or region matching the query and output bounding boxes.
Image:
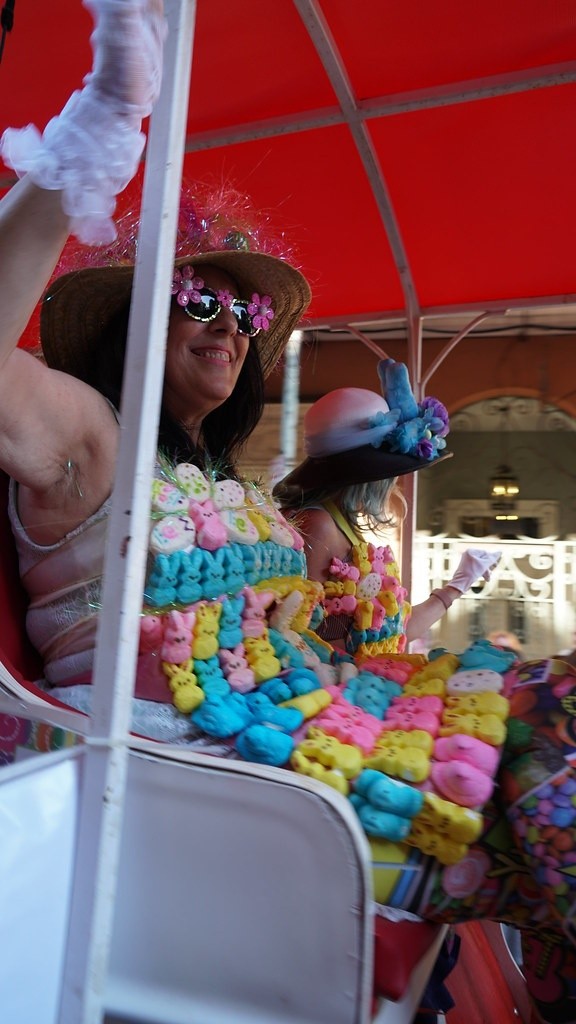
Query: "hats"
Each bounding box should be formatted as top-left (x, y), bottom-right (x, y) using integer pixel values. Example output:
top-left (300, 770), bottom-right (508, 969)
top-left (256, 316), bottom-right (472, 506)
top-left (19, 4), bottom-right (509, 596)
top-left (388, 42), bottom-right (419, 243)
top-left (272, 359), bottom-right (454, 503)
top-left (39, 188), bottom-right (312, 383)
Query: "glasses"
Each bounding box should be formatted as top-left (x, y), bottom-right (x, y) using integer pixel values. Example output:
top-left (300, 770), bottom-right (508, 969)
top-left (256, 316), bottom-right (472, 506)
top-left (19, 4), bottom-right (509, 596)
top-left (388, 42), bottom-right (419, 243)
top-left (171, 265), bottom-right (274, 337)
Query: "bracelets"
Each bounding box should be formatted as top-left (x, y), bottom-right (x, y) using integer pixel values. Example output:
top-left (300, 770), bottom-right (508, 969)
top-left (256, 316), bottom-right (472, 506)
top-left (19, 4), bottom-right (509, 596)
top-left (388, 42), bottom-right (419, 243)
top-left (430, 587), bottom-right (452, 608)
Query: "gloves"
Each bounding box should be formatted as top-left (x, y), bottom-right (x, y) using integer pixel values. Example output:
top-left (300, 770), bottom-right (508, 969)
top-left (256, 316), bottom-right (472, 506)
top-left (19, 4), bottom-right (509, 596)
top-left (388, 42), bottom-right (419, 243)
top-left (444, 548), bottom-right (502, 594)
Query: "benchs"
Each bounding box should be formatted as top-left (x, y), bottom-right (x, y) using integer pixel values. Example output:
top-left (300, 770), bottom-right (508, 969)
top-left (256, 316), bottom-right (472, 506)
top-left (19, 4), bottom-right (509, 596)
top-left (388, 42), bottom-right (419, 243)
top-left (0, 535), bottom-right (454, 1024)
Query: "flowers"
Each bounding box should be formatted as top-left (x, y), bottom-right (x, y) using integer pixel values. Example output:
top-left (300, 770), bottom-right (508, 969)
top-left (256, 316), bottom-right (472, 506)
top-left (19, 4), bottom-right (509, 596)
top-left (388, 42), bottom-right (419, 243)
top-left (416, 422), bottom-right (440, 461)
top-left (247, 291), bottom-right (275, 331)
top-left (172, 265), bottom-right (205, 306)
top-left (217, 289), bottom-right (235, 307)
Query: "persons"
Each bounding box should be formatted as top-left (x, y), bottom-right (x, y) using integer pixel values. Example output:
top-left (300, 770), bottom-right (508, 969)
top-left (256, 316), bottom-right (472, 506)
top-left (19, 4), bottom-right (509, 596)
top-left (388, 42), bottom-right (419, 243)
top-left (487, 630), bottom-right (520, 650)
top-left (0, 0), bottom-right (576, 1024)
top-left (236, 310), bottom-right (251, 333)
top-left (272, 388), bottom-right (502, 654)
top-left (191, 299), bottom-right (215, 318)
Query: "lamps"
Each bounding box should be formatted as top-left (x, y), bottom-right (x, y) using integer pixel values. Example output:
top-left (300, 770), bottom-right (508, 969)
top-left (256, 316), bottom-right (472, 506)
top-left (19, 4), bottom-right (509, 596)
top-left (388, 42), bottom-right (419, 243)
top-left (488, 450), bottom-right (520, 510)
